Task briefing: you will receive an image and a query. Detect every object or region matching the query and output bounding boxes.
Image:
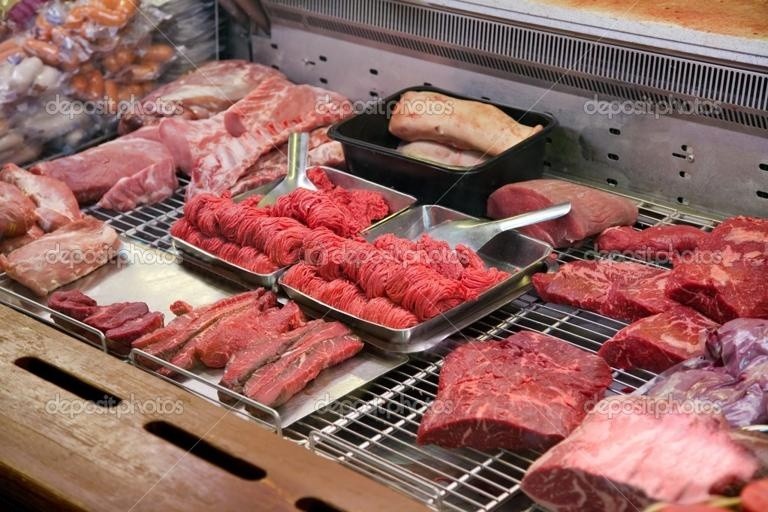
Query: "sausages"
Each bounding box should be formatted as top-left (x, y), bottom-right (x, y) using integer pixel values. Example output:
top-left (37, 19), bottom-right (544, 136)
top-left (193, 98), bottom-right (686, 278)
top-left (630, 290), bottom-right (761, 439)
top-left (0, 0), bottom-right (177, 171)
